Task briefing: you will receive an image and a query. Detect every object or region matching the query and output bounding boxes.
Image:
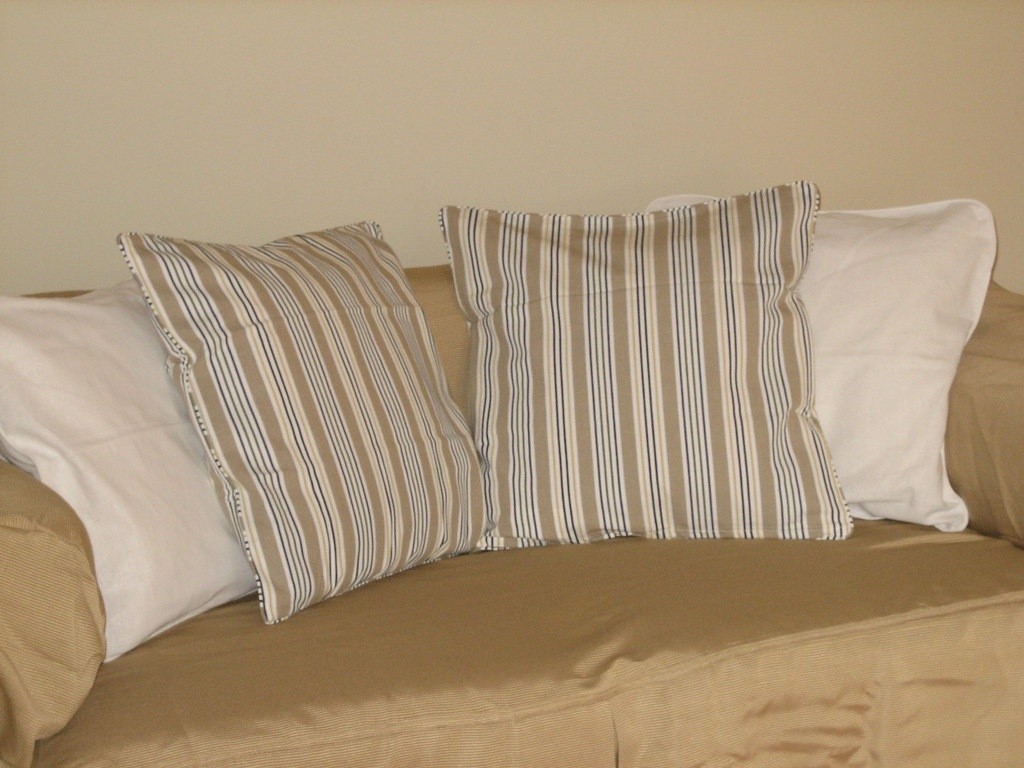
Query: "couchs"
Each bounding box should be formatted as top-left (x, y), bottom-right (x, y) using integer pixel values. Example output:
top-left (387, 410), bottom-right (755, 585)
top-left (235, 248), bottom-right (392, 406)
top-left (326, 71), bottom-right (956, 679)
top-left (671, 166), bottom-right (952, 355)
top-left (0, 265), bottom-right (1024, 768)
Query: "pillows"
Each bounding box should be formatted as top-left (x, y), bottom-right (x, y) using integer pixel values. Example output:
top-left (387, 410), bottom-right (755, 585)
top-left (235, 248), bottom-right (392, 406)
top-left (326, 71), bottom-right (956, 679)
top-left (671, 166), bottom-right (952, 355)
top-left (0, 277), bottom-right (256, 666)
top-left (115, 222), bottom-right (490, 627)
top-left (644, 193), bottom-right (998, 534)
top-left (431, 179), bottom-right (857, 544)
